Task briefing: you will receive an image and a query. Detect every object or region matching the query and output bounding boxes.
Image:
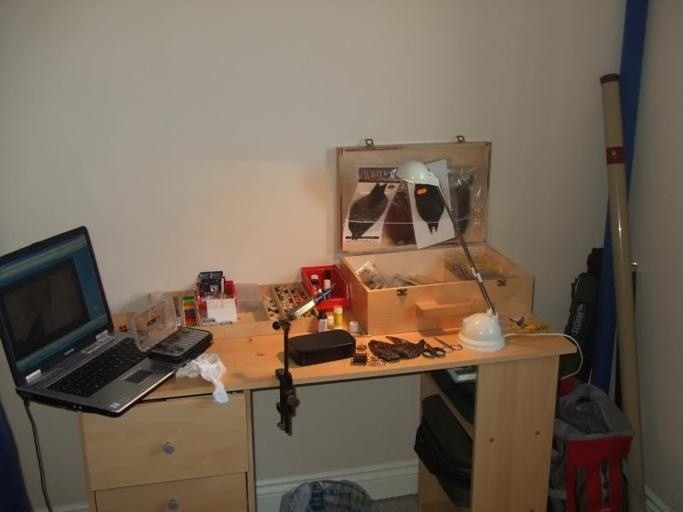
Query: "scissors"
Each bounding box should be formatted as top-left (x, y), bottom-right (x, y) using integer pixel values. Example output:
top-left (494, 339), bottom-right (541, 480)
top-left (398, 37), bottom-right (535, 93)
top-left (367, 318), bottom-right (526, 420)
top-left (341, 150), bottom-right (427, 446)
top-left (434, 337), bottom-right (463, 353)
top-left (421, 339), bottom-right (446, 358)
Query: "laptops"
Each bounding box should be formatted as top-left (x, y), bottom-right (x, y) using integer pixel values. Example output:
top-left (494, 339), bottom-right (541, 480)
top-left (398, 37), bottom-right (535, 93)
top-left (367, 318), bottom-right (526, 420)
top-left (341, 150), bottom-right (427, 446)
top-left (0, 226), bottom-right (214, 418)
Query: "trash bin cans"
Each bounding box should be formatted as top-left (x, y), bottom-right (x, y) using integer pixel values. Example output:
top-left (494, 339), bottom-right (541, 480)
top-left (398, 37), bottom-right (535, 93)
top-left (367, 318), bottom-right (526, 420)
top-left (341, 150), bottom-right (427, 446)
top-left (288, 479), bottom-right (379, 512)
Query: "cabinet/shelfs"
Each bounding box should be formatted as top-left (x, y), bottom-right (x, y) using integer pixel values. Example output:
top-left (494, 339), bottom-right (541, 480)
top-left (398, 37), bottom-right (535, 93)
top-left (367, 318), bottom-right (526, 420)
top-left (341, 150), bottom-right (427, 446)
top-left (418, 356), bottom-right (561, 512)
top-left (81, 390), bottom-right (257, 512)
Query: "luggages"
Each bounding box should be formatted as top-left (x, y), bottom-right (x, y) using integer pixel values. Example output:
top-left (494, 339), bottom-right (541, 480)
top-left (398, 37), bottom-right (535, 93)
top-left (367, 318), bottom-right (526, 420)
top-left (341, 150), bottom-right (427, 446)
top-left (414, 394), bottom-right (473, 506)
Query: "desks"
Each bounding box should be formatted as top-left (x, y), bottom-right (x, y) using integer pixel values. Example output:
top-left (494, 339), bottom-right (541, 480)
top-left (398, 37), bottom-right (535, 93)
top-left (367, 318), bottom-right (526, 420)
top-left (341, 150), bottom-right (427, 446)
top-left (75, 310), bottom-right (578, 512)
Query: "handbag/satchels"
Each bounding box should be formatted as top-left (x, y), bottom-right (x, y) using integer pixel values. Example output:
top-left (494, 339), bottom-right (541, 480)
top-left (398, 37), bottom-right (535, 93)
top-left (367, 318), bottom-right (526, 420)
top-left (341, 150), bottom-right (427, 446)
top-left (546, 374), bottom-right (633, 510)
